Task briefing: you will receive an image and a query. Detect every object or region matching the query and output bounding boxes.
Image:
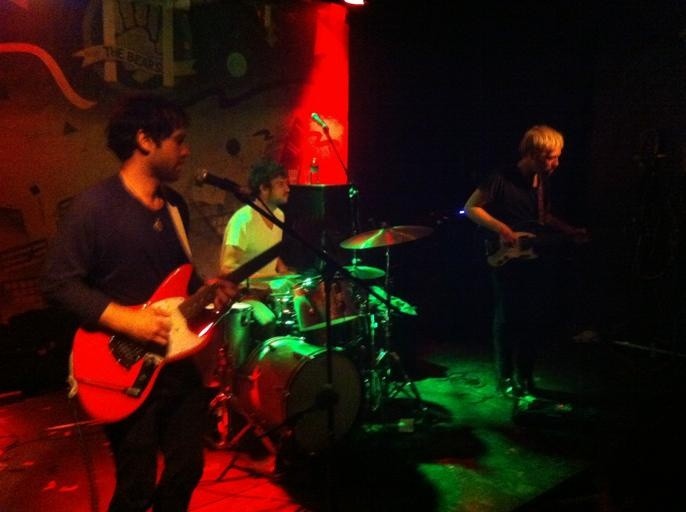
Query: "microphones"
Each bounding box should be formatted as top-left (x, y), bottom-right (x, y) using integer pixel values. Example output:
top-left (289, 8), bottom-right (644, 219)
top-left (293, 387), bottom-right (340, 419)
top-left (312, 111), bottom-right (328, 132)
top-left (192, 167), bottom-right (239, 193)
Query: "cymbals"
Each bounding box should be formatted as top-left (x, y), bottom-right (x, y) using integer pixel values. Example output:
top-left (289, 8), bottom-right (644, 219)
top-left (241, 274), bottom-right (299, 282)
top-left (342, 265), bottom-right (387, 280)
top-left (340, 222), bottom-right (436, 250)
top-left (370, 285), bottom-right (418, 316)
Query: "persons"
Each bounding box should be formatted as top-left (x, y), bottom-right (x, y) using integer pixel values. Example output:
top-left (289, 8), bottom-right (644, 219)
top-left (220, 160), bottom-right (300, 283)
top-left (463, 123), bottom-right (593, 401)
top-left (35, 90), bottom-right (241, 510)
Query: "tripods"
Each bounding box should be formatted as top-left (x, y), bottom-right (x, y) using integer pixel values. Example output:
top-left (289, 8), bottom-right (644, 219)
top-left (357, 320), bottom-right (424, 420)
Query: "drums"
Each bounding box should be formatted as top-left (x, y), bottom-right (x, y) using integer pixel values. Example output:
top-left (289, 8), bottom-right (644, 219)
top-left (292, 270), bottom-right (366, 330)
top-left (232, 335), bottom-right (364, 453)
top-left (205, 301), bottom-right (252, 367)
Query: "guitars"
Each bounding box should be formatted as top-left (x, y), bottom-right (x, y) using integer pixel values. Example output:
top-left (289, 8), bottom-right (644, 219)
top-left (484, 221), bottom-right (591, 267)
top-left (67, 233), bottom-right (303, 426)
